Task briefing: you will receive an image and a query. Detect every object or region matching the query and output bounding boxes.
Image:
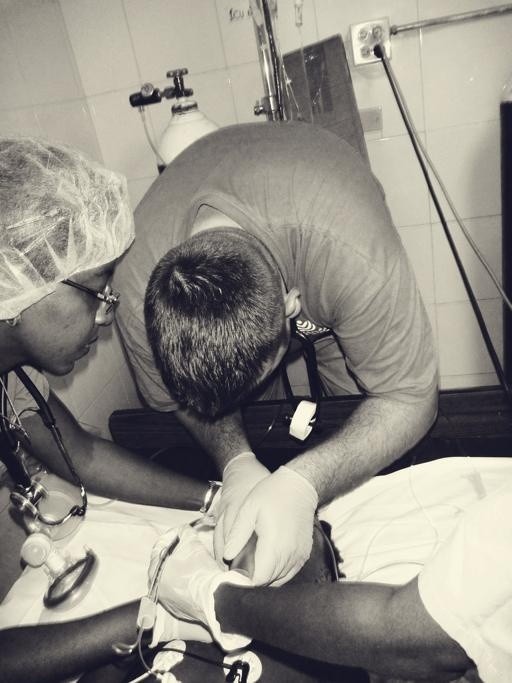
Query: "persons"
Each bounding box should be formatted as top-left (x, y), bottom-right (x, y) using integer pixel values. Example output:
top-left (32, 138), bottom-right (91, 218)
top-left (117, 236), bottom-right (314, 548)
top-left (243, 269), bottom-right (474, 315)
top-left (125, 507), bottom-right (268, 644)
top-left (0, 135), bottom-right (224, 681)
top-left (147, 512), bottom-right (512, 683)
top-left (109, 118), bottom-right (442, 591)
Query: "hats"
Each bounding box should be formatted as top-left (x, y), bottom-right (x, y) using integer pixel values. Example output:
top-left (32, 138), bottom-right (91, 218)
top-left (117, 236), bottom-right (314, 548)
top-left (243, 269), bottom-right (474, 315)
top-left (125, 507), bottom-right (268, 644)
top-left (1, 138), bottom-right (138, 322)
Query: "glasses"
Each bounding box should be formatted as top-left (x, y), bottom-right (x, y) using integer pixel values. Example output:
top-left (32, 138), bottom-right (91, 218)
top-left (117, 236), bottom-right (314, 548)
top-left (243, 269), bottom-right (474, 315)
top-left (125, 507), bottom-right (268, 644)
top-left (65, 275), bottom-right (119, 329)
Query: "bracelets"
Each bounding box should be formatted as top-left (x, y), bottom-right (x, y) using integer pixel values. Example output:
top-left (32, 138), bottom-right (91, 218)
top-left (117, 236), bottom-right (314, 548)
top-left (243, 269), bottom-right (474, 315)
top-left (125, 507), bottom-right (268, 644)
top-left (198, 478), bottom-right (218, 513)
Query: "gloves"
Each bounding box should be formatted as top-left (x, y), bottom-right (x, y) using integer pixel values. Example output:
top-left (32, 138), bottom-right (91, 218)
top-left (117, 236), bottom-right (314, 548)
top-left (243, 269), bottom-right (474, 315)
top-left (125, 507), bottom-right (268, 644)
top-left (146, 523), bottom-right (243, 650)
top-left (212, 453), bottom-right (318, 588)
